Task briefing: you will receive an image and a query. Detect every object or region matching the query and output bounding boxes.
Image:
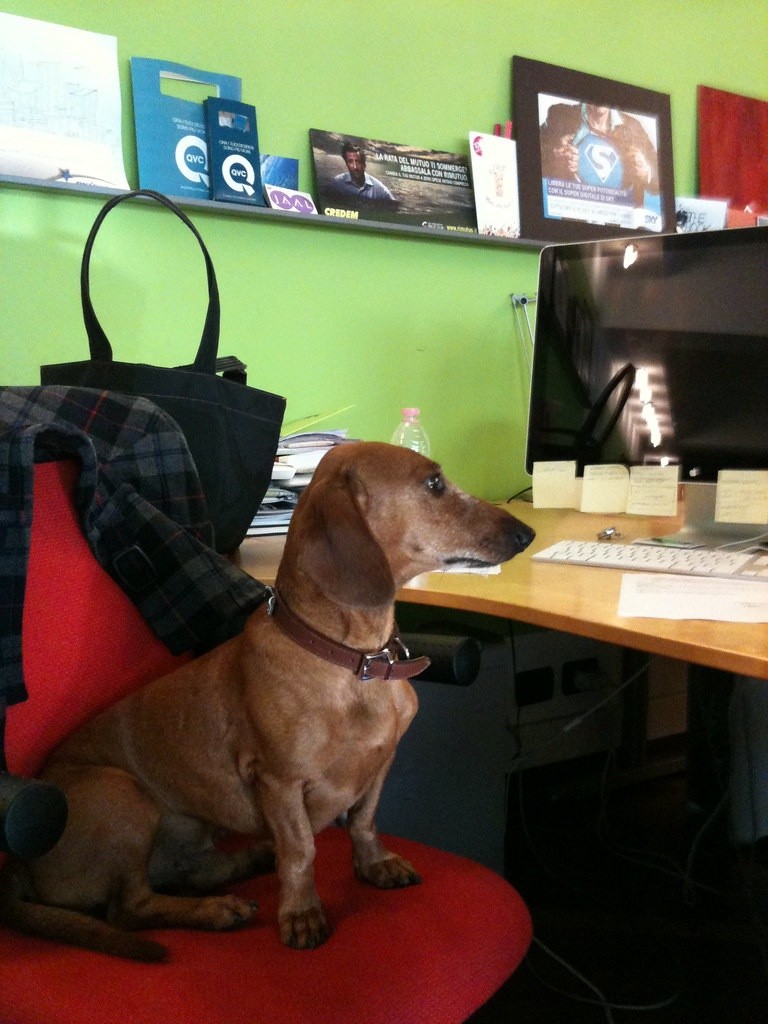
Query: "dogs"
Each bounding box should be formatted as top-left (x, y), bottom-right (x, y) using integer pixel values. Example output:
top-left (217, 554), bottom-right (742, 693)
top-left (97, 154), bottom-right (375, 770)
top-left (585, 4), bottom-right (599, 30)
top-left (0, 441), bottom-right (537, 963)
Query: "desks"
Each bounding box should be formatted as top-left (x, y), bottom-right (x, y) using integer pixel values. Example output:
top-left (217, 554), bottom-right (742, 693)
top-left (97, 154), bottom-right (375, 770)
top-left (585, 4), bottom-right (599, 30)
top-left (213, 501), bottom-right (768, 905)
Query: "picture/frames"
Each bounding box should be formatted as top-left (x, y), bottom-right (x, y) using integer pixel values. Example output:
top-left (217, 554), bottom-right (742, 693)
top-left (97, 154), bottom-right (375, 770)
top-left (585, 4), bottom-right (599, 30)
top-left (513, 54), bottom-right (676, 243)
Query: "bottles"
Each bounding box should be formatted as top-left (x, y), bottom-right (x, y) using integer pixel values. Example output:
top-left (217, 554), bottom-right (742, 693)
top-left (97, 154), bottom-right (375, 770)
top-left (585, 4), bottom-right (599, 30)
top-left (389, 407), bottom-right (430, 459)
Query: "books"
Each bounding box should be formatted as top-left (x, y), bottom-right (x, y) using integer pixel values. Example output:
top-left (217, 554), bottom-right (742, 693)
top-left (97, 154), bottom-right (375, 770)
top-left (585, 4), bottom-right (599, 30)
top-left (246, 430), bottom-right (343, 537)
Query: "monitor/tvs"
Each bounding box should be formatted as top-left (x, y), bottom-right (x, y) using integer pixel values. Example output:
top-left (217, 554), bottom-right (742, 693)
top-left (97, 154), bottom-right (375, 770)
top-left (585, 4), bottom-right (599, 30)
top-left (524, 226), bottom-right (768, 555)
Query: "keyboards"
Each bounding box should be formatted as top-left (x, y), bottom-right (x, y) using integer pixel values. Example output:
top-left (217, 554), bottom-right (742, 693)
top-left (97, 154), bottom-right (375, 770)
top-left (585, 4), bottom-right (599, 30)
top-left (530, 540), bottom-right (768, 584)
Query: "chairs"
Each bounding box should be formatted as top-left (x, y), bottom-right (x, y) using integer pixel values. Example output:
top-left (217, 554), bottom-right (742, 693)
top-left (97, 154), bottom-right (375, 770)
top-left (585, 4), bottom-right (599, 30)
top-left (0, 452), bottom-right (535, 1024)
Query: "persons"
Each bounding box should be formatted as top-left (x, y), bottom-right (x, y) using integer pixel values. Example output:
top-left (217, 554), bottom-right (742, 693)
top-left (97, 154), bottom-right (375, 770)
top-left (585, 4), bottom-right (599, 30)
top-left (540, 100), bottom-right (662, 209)
top-left (332, 141), bottom-right (395, 202)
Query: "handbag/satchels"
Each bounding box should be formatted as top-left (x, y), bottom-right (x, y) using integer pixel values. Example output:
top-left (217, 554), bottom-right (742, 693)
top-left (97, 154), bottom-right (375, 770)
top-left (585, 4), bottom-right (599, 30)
top-left (40, 190), bottom-right (286, 560)
